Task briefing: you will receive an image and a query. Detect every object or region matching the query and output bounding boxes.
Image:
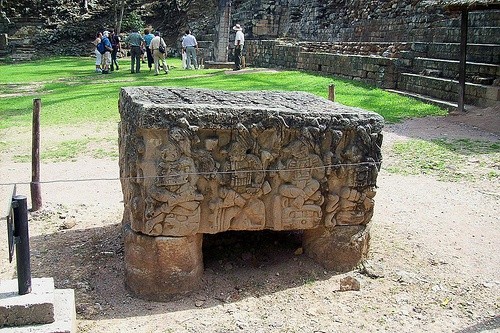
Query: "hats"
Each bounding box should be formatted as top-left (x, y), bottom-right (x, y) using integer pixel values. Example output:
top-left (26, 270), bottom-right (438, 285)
top-left (103, 31), bottom-right (111, 36)
top-left (233, 24), bottom-right (243, 31)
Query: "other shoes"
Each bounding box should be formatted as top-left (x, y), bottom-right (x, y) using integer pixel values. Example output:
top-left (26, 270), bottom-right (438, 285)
top-left (111, 68), bottom-right (114, 71)
top-left (165, 71), bottom-right (168, 74)
top-left (142, 61), bottom-right (146, 64)
top-left (159, 68), bottom-right (163, 70)
top-left (131, 71), bottom-right (135, 74)
top-left (136, 70), bottom-right (140, 73)
top-left (105, 70), bottom-right (112, 73)
top-left (116, 67), bottom-right (119, 70)
top-left (154, 71), bottom-right (159, 76)
top-left (95, 68), bottom-right (102, 72)
top-left (236, 68), bottom-right (240, 70)
top-left (102, 70), bottom-right (105, 74)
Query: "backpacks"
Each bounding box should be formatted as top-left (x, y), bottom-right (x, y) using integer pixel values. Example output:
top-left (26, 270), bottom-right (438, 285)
top-left (97, 38), bottom-right (110, 54)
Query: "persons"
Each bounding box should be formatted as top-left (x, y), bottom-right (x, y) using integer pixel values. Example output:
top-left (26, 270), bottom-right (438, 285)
top-left (93, 28), bottom-right (121, 74)
top-left (183, 30), bottom-right (198, 70)
top-left (125, 28), bottom-right (169, 76)
top-left (233, 24), bottom-right (244, 71)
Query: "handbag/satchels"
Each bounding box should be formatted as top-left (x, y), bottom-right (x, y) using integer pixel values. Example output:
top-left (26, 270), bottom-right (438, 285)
top-left (159, 45), bottom-right (165, 53)
